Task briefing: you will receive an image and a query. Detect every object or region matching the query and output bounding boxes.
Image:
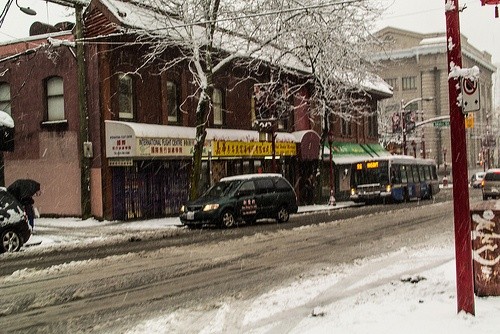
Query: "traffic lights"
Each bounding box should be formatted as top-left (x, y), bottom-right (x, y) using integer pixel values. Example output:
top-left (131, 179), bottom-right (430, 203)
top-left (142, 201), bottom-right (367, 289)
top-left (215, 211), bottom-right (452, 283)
top-left (403, 109), bottom-right (412, 131)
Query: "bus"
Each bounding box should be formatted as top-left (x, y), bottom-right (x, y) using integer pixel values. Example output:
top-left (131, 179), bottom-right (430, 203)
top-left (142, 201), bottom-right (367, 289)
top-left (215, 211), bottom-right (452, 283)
top-left (349, 156), bottom-right (440, 205)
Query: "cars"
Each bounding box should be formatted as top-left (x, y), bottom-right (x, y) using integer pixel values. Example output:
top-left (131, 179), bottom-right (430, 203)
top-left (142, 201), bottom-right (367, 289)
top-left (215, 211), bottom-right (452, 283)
top-left (472, 172), bottom-right (487, 189)
top-left (0, 187), bottom-right (34, 254)
top-left (179, 174), bottom-right (299, 229)
top-left (482, 168), bottom-right (500, 200)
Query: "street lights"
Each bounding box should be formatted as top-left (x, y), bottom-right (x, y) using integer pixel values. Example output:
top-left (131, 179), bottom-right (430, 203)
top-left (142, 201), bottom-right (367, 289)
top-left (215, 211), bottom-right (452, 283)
top-left (399, 96), bottom-right (433, 158)
